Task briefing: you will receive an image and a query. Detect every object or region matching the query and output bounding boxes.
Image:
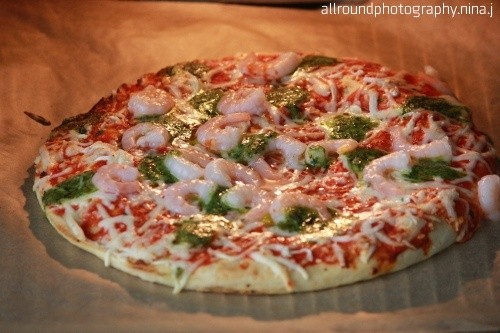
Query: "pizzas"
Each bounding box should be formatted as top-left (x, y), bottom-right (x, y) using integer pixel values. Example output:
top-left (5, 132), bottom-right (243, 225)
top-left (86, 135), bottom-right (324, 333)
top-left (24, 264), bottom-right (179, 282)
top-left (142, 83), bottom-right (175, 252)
top-left (35, 51), bottom-right (496, 297)
